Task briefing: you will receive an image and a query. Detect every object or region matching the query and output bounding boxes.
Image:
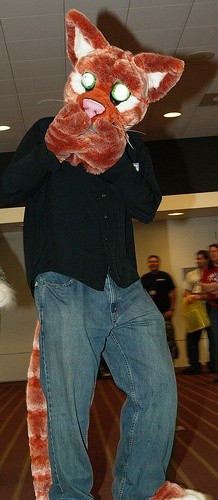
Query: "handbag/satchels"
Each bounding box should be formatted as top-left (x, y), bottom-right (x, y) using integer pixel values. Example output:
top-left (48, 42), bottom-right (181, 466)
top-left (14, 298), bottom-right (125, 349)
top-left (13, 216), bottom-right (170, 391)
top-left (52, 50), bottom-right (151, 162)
top-left (180, 296), bottom-right (211, 333)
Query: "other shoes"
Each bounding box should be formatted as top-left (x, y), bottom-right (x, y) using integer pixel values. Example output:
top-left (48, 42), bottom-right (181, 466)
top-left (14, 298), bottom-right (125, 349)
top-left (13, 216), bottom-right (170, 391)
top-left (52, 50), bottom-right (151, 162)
top-left (206, 362), bottom-right (218, 373)
top-left (180, 365), bottom-right (201, 375)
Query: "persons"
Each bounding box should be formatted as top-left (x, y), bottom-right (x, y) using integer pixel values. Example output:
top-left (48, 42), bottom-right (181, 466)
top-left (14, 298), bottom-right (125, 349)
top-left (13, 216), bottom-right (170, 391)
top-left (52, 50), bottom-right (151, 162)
top-left (0, 11), bottom-right (208, 500)
top-left (99, 244), bottom-right (218, 378)
